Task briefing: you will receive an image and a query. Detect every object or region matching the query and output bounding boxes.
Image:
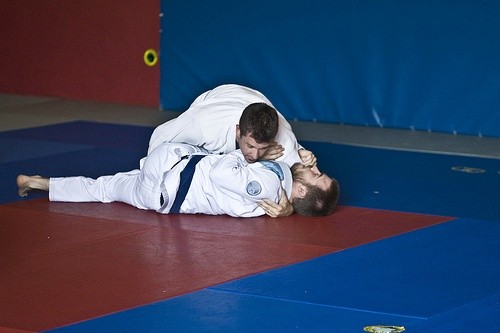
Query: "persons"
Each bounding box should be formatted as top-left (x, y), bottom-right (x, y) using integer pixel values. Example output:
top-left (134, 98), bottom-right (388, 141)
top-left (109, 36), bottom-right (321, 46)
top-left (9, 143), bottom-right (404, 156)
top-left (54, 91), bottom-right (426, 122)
top-left (16, 140), bottom-right (340, 219)
top-left (139, 82), bottom-right (299, 219)
